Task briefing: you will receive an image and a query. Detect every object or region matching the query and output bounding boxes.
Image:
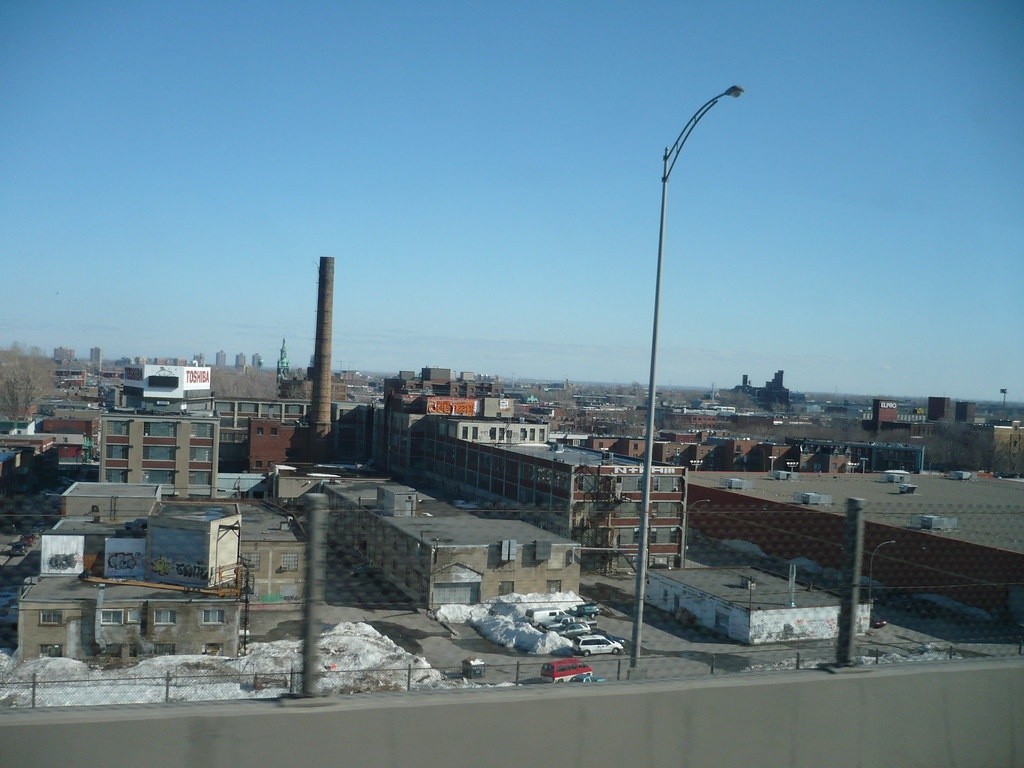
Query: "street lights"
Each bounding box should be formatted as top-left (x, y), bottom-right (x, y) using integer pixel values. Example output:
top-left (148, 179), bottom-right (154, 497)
top-left (628, 85), bottom-right (745, 659)
top-left (684, 499), bottom-right (711, 568)
top-left (868, 540), bottom-right (897, 602)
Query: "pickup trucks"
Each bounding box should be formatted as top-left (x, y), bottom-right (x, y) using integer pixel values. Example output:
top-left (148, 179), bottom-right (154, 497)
top-left (538, 615), bottom-right (598, 633)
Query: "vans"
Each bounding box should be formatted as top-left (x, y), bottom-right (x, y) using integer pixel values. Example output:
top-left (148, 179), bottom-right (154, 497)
top-left (525, 608), bottom-right (570, 627)
top-left (541, 658), bottom-right (594, 684)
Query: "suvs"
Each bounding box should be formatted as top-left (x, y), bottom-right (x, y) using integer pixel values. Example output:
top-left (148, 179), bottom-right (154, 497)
top-left (573, 635), bottom-right (624, 657)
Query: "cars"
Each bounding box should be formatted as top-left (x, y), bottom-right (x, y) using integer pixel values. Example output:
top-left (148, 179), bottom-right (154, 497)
top-left (583, 630), bottom-right (626, 647)
top-left (569, 674), bottom-right (605, 683)
top-left (10, 528), bottom-right (41, 558)
top-left (870, 613), bottom-right (887, 628)
top-left (564, 604), bottom-right (601, 619)
top-left (550, 623), bottom-right (593, 639)
